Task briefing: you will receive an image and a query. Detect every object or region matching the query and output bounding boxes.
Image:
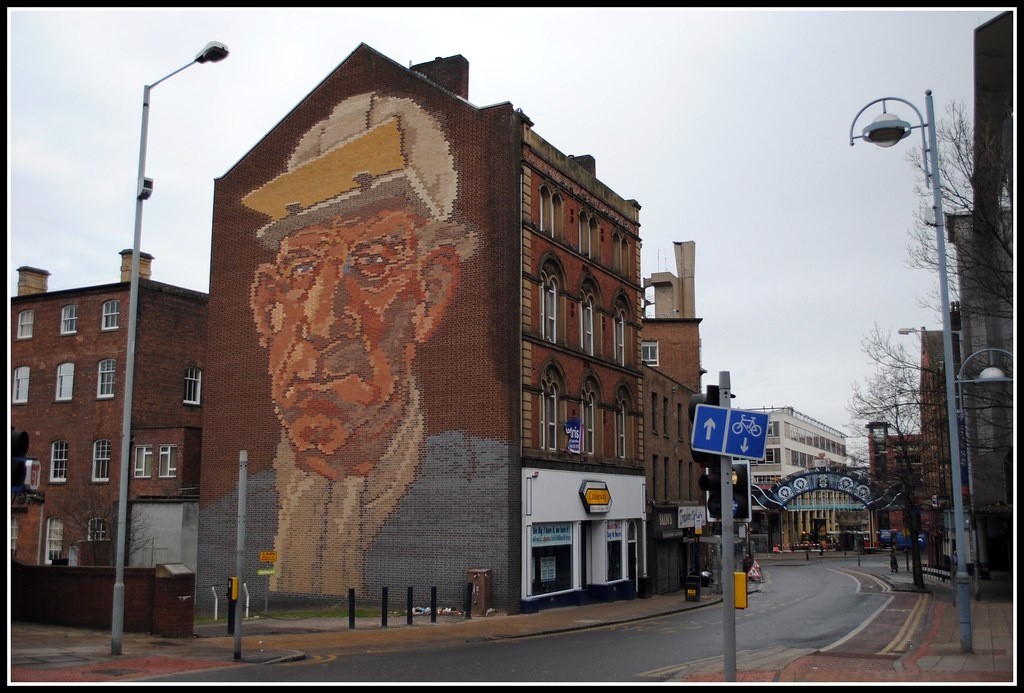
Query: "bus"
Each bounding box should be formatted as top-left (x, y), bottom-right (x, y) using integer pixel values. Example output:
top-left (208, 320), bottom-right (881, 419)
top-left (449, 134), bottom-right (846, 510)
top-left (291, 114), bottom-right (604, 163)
top-left (825, 530), bottom-right (880, 553)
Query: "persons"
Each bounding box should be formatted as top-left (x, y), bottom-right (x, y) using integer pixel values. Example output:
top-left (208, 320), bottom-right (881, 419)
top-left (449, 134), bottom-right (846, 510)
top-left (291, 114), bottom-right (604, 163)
top-left (819, 543), bottom-right (824, 556)
top-left (743, 554), bottom-right (752, 574)
top-left (890, 550), bottom-right (898, 573)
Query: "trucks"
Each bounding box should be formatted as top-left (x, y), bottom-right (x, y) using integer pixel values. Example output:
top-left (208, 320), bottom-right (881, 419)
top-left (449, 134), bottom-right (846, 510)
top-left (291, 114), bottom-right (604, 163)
top-left (879, 529), bottom-right (892, 547)
top-left (892, 528), bottom-right (927, 551)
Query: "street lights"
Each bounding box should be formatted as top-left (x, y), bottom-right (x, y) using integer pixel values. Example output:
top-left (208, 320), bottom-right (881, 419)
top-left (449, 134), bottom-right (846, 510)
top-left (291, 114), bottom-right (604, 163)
top-left (896, 328), bottom-right (982, 601)
top-left (109, 41), bottom-right (229, 657)
top-left (849, 90), bottom-right (1012, 655)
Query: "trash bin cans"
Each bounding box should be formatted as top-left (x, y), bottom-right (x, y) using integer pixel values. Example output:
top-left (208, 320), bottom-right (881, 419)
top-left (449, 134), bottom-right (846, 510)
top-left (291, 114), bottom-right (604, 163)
top-left (466, 569), bottom-right (493, 617)
top-left (685, 576), bottom-right (700, 602)
top-left (150, 563), bottom-right (198, 639)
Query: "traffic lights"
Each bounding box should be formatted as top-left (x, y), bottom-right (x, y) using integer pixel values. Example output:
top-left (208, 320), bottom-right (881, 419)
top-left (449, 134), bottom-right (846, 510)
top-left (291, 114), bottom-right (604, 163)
top-left (688, 384), bottom-right (722, 468)
top-left (11, 426), bottom-right (29, 491)
top-left (698, 473), bottom-right (722, 520)
top-left (732, 461), bottom-right (750, 518)
